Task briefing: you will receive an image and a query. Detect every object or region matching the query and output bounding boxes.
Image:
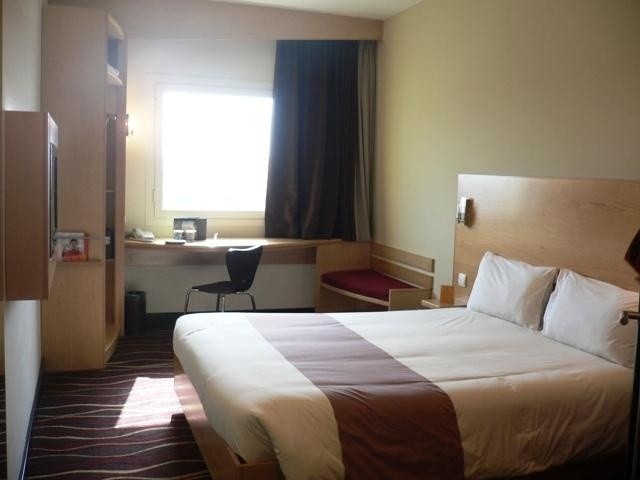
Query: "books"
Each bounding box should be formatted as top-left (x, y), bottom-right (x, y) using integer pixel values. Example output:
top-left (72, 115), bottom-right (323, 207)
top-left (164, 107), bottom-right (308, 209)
top-left (165, 239), bottom-right (186, 245)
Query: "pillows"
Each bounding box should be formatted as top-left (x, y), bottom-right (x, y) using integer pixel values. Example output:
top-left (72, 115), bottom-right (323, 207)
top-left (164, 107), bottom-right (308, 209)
top-left (540, 268), bottom-right (639, 371)
top-left (467, 250), bottom-right (559, 332)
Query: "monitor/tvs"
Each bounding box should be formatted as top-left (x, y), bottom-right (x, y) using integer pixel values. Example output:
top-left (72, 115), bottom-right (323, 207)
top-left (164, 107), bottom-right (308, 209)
top-left (50, 142), bottom-right (58, 263)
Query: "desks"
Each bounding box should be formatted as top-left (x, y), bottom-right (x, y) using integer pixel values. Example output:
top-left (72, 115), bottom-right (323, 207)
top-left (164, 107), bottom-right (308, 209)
top-left (125, 235), bottom-right (343, 267)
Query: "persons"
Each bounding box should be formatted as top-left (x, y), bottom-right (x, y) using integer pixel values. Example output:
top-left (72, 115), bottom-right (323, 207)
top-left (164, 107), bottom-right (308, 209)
top-left (62, 239), bottom-right (83, 256)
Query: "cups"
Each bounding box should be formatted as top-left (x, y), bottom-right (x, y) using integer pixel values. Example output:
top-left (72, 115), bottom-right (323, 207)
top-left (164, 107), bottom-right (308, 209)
top-left (173, 229), bottom-right (183, 240)
top-left (184, 230), bottom-right (197, 242)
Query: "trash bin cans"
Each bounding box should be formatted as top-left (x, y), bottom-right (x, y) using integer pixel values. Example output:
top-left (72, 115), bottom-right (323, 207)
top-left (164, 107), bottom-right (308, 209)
top-left (125, 290), bottom-right (146, 336)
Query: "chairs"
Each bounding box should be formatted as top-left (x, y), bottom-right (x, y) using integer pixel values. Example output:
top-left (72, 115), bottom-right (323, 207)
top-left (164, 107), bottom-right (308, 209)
top-left (184, 245), bottom-right (263, 314)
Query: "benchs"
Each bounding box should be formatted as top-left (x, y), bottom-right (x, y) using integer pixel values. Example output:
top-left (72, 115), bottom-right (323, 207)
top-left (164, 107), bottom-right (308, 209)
top-left (314, 241), bottom-right (434, 313)
top-left (175, 175), bottom-right (640, 480)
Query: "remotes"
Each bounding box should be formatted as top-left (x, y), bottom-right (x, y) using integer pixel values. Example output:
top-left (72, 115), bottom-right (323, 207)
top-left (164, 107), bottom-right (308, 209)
top-left (166, 239), bottom-right (186, 244)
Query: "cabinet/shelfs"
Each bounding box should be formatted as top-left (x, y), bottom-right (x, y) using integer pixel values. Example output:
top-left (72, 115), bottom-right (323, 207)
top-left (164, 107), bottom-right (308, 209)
top-left (41, 5), bottom-right (127, 371)
top-left (5, 111), bottom-right (60, 299)
